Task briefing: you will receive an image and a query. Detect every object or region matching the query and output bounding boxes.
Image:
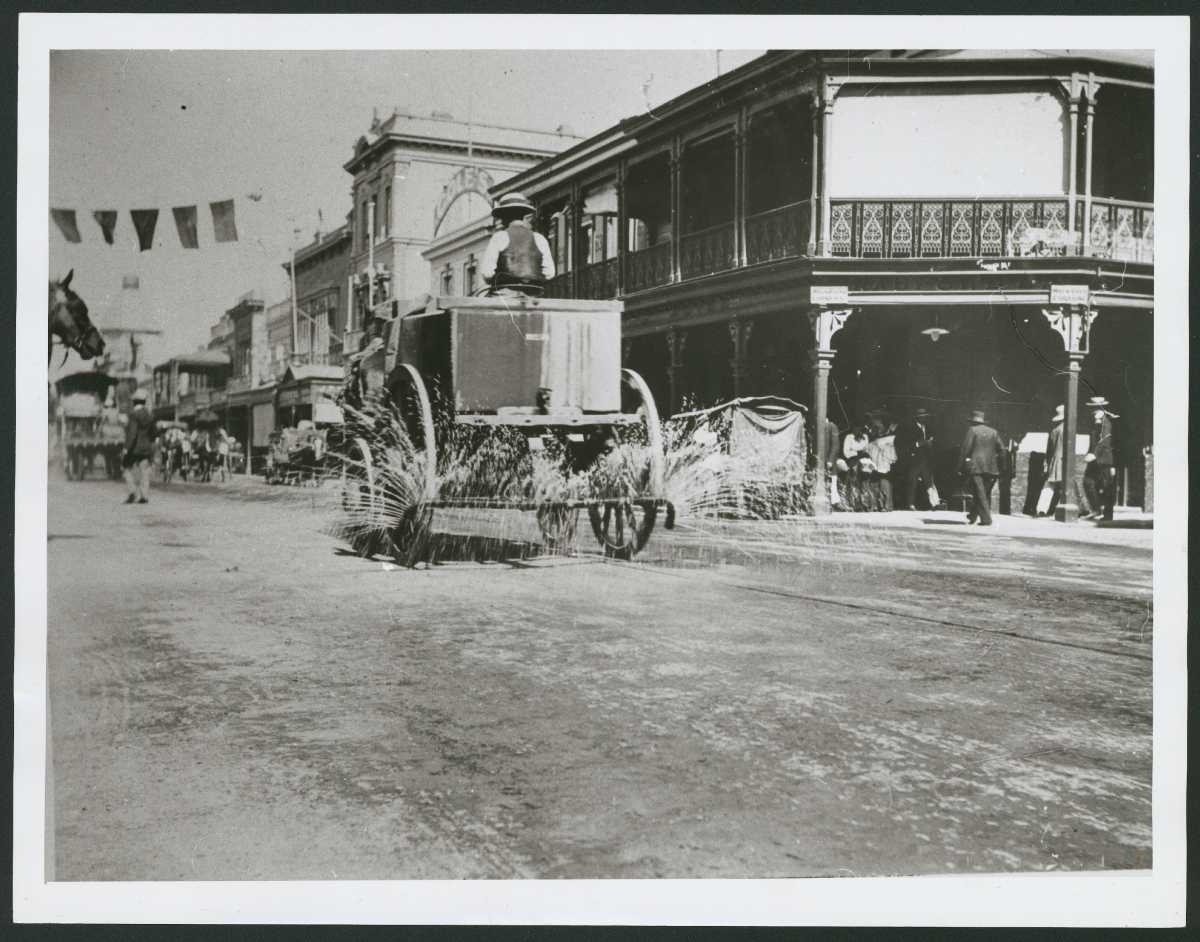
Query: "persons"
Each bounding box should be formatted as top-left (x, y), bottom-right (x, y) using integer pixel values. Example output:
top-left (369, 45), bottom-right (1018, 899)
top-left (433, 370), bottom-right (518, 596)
top-left (952, 409), bottom-right (1008, 525)
top-left (1045, 404), bottom-right (1065, 490)
top-left (479, 194), bottom-right (556, 298)
top-left (165, 424), bottom-right (233, 481)
top-left (1081, 394), bottom-right (1119, 521)
top-left (116, 389), bottom-right (159, 503)
top-left (825, 405), bottom-right (939, 512)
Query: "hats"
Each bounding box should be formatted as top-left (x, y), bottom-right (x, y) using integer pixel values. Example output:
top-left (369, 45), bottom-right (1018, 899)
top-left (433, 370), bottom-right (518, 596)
top-left (915, 408), bottom-right (929, 419)
top-left (131, 388), bottom-right (148, 404)
top-left (490, 192), bottom-right (539, 218)
top-left (1086, 395), bottom-right (1111, 408)
top-left (1053, 404), bottom-right (1066, 423)
top-left (966, 410), bottom-right (986, 426)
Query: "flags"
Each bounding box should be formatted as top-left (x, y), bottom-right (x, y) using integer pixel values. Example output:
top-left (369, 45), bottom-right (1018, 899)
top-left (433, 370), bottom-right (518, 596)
top-left (172, 205), bottom-right (199, 249)
top-left (51, 209), bottom-right (82, 243)
top-left (210, 199), bottom-right (239, 242)
top-left (129, 209), bottom-right (158, 251)
top-left (96, 211), bottom-right (117, 244)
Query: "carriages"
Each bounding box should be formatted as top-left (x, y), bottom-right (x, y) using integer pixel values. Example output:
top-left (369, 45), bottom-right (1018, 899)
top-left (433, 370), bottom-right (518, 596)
top-left (153, 352), bottom-right (237, 482)
top-left (316, 292), bottom-right (685, 564)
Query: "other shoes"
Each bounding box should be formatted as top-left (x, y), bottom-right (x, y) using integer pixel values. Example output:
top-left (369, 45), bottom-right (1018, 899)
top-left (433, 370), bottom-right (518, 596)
top-left (139, 497), bottom-right (148, 504)
top-left (966, 514), bottom-right (976, 524)
top-left (976, 520), bottom-right (992, 525)
top-left (125, 494), bottom-right (138, 503)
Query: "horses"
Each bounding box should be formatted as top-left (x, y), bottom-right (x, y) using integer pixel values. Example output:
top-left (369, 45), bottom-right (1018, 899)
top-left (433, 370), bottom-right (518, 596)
top-left (342, 300), bottom-right (399, 508)
top-left (287, 431), bottom-right (325, 487)
top-left (49, 268), bottom-right (107, 363)
top-left (195, 428), bottom-right (231, 483)
